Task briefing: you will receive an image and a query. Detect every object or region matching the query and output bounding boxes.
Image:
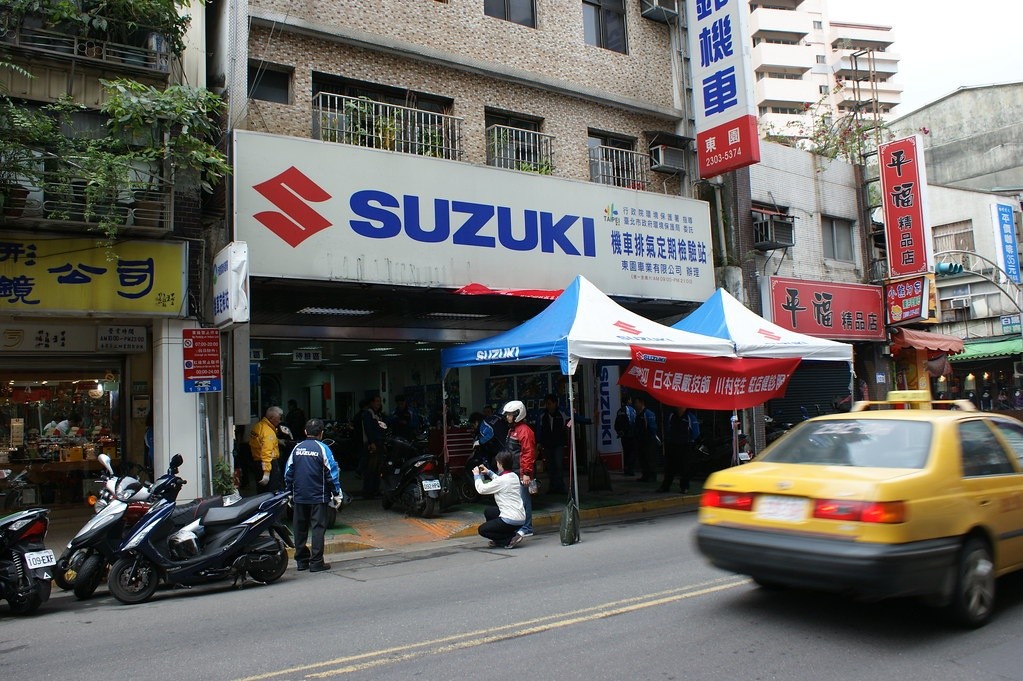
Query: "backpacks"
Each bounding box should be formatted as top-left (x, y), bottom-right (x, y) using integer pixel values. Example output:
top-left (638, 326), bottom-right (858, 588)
top-left (615, 405), bottom-right (629, 438)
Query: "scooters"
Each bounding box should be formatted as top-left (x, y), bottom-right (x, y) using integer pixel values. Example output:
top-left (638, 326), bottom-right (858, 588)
top-left (278, 423), bottom-right (352, 529)
top-left (377, 419), bottom-right (443, 519)
top-left (54, 452), bottom-right (294, 605)
top-left (0, 507), bottom-right (56, 617)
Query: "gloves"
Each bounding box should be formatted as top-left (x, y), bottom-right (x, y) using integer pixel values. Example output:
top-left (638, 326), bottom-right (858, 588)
top-left (287, 495), bottom-right (294, 507)
top-left (329, 488), bottom-right (342, 509)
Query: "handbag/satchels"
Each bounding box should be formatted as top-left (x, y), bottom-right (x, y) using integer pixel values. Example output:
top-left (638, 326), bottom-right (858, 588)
top-left (587, 453), bottom-right (612, 493)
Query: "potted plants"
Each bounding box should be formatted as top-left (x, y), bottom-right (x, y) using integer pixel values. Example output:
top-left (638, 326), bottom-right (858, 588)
top-left (95, 75), bottom-right (232, 227)
top-left (0, 101), bottom-right (64, 216)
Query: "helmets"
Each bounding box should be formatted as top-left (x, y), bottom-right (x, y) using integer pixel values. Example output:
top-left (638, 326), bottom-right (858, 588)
top-left (501, 400), bottom-right (527, 423)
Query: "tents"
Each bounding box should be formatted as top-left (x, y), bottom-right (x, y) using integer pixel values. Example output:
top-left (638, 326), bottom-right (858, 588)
top-left (441, 275), bottom-right (855, 508)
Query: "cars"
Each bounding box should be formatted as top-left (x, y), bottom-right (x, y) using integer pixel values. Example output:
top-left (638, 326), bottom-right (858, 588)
top-left (695, 390), bottom-right (1023, 630)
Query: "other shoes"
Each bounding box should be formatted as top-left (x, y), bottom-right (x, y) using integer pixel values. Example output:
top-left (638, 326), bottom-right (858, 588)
top-left (657, 485), bottom-right (665, 492)
top-left (679, 488), bottom-right (688, 494)
top-left (518, 531), bottom-right (534, 537)
top-left (363, 492), bottom-right (372, 499)
top-left (372, 492), bottom-right (383, 499)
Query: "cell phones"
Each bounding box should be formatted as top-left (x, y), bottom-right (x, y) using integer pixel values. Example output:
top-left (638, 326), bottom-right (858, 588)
top-left (478, 467), bottom-right (483, 473)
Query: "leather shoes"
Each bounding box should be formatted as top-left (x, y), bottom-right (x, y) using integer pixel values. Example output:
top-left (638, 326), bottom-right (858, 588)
top-left (310, 563), bottom-right (330, 572)
top-left (297, 561), bottom-right (309, 571)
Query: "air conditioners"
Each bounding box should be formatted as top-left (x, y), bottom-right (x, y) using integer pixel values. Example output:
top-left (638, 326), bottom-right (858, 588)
top-left (951, 298), bottom-right (968, 309)
top-left (649, 145), bottom-right (687, 174)
top-left (640, 0), bottom-right (679, 22)
top-left (752, 220), bottom-right (796, 245)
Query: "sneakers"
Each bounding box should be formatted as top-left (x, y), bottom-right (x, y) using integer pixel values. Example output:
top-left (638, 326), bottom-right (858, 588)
top-left (488, 541), bottom-right (502, 547)
top-left (505, 533), bottom-right (522, 549)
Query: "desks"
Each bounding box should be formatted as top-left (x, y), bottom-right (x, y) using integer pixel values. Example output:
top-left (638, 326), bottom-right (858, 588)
top-left (13, 459), bottom-right (122, 493)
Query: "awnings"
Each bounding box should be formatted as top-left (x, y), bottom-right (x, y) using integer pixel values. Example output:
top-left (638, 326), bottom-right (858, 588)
top-left (948, 340), bottom-right (1023, 360)
top-left (891, 327), bottom-right (963, 360)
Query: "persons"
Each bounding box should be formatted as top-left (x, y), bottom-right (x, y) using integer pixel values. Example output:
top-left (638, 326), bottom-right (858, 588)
top-left (964, 371), bottom-right (1023, 411)
top-left (617, 393), bottom-right (638, 476)
top-left (502, 400), bottom-right (536, 537)
top-left (936, 375), bottom-right (947, 394)
top-left (56, 414), bottom-right (82, 435)
top-left (249, 405), bottom-right (284, 494)
top-left (44, 416), bottom-right (62, 431)
top-left (655, 404), bottom-right (700, 494)
top-left (283, 418), bottom-right (343, 572)
top-left (286, 400), bottom-right (307, 441)
top-left (633, 397), bottom-right (658, 483)
top-left (540, 394), bottom-right (567, 497)
top-left (470, 412), bottom-right (505, 468)
top-left (352, 394), bottom-right (421, 500)
top-left (472, 451), bottom-right (527, 549)
